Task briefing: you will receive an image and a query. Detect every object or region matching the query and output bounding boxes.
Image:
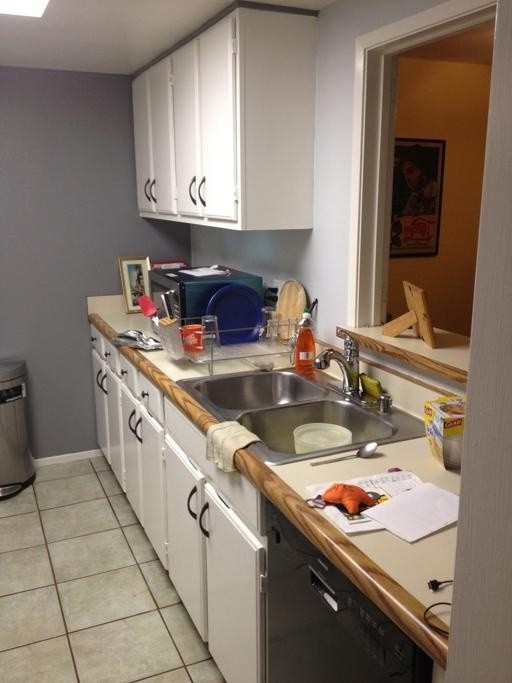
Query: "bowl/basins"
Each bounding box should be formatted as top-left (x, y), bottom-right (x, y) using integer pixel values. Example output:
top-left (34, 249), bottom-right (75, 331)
top-left (293, 420), bottom-right (354, 454)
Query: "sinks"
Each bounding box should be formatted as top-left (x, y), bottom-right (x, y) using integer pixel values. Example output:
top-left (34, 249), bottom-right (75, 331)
top-left (235, 400), bottom-right (427, 466)
top-left (175, 365), bottom-right (342, 409)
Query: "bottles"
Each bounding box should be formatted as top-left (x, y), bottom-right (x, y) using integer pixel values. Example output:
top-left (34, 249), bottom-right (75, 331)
top-left (294, 328), bottom-right (317, 378)
top-left (159, 317), bottom-right (186, 361)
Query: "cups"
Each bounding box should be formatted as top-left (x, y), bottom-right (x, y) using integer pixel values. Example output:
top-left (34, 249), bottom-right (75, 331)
top-left (201, 315), bottom-right (222, 347)
top-left (181, 323), bottom-right (204, 352)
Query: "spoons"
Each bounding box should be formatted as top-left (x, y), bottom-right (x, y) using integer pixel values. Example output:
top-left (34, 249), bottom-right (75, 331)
top-left (310, 441), bottom-right (380, 466)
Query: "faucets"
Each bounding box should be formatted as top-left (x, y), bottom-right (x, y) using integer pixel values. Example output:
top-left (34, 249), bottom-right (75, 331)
top-left (312, 328), bottom-right (359, 396)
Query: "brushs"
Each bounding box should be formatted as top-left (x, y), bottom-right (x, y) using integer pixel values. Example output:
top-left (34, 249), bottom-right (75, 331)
top-left (138, 295), bottom-right (160, 327)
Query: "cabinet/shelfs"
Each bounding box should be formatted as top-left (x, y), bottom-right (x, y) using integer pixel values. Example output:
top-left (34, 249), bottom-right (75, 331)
top-left (132, 56), bottom-right (177, 216)
top-left (89, 323), bottom-right (125, 493)
top-left (163, 395), bottom-right (264, 683)
top-left (119, 351), bottom-right (167, 570)
top-left (172, 16), bottom-right (315, 222)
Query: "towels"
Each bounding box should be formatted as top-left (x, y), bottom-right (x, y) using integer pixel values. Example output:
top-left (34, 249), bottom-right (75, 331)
top-left (205, 421), bottom-right (266, 472)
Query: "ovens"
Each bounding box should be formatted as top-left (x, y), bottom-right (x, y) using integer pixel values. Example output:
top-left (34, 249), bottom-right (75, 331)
top-left (263, 498), bottom-right (435, 682)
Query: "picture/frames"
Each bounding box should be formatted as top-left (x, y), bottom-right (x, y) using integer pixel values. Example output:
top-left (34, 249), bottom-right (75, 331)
top-left (381, 280), bottom-right (438, 349)
top-left (118, 256), bottom-right (154, 314)
top-left (390, 137), bottom-right (446, 258)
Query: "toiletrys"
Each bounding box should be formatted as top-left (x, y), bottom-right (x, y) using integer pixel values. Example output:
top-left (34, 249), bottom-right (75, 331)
top-left (295, 297), bottom-right (320, 374)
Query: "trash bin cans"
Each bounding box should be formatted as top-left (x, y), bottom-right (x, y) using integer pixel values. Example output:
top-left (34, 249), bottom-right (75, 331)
top-left (0, 359), bottom-right (37, 501)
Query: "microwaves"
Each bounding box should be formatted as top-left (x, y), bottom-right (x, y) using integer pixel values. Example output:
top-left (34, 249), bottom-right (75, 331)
top-left (148, 265), bottom-right (263, 337)
